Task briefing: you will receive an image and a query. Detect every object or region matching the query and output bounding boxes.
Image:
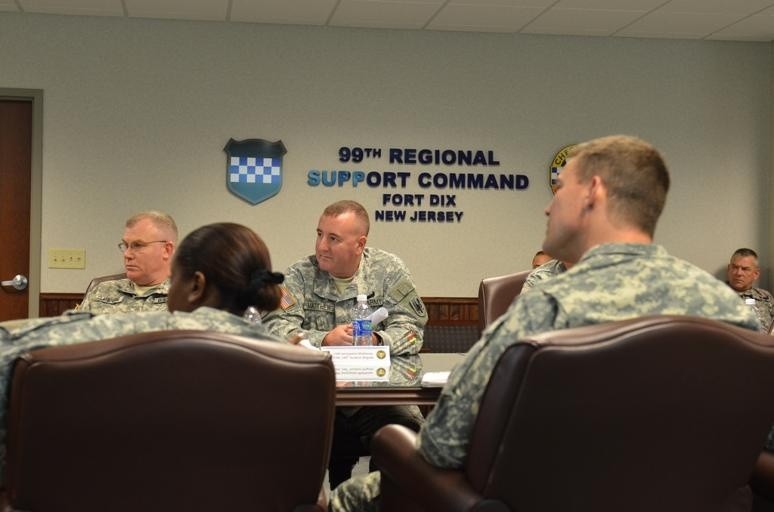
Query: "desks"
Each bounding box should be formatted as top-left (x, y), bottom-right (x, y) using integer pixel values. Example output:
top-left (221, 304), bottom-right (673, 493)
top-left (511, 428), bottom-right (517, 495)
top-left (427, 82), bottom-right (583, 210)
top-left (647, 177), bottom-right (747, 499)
top-left (315, 351), bottom-right (469, 406)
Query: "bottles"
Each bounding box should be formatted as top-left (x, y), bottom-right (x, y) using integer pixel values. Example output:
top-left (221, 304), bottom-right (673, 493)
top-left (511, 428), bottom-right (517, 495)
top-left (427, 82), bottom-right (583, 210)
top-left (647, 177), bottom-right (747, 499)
top-left (352, 295), bottom-right (370, 348)
top-left (744, 298), bottom-right (761, 321)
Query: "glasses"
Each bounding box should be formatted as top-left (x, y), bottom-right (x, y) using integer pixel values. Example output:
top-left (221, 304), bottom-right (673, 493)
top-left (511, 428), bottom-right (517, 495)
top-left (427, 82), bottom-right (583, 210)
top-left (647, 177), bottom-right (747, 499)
top-left (118, 241), bottom-right (166, 251)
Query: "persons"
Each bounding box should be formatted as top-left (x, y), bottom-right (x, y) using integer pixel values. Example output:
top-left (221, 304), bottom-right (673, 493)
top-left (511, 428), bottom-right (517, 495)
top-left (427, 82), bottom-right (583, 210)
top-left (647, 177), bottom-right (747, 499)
top-left (75, 211), bottom-right (179, 316)
top-left (326, 135), bottom-right (760, 511)
top-left (258, 199), bottom-right (429, 490)
top-left (519, 259), bottom-right (574, 292)
top-left (724, 247), bottom-right (773, 334)
top-left (532, 250), bottom-right (552, 269)
top-left (0, 220), bottom-right (332, 508)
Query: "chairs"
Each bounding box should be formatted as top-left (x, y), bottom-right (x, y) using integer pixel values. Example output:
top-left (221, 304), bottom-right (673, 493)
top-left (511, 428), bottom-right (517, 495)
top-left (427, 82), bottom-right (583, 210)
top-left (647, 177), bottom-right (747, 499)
top-left (477, 270), bottom-right (533, 335)
top-left (371, 314), bottom-right (773, 511)
top-left (85, 273), bottom-right (128, 295)
top-left (0, 329), bottom-right (335, 512)
